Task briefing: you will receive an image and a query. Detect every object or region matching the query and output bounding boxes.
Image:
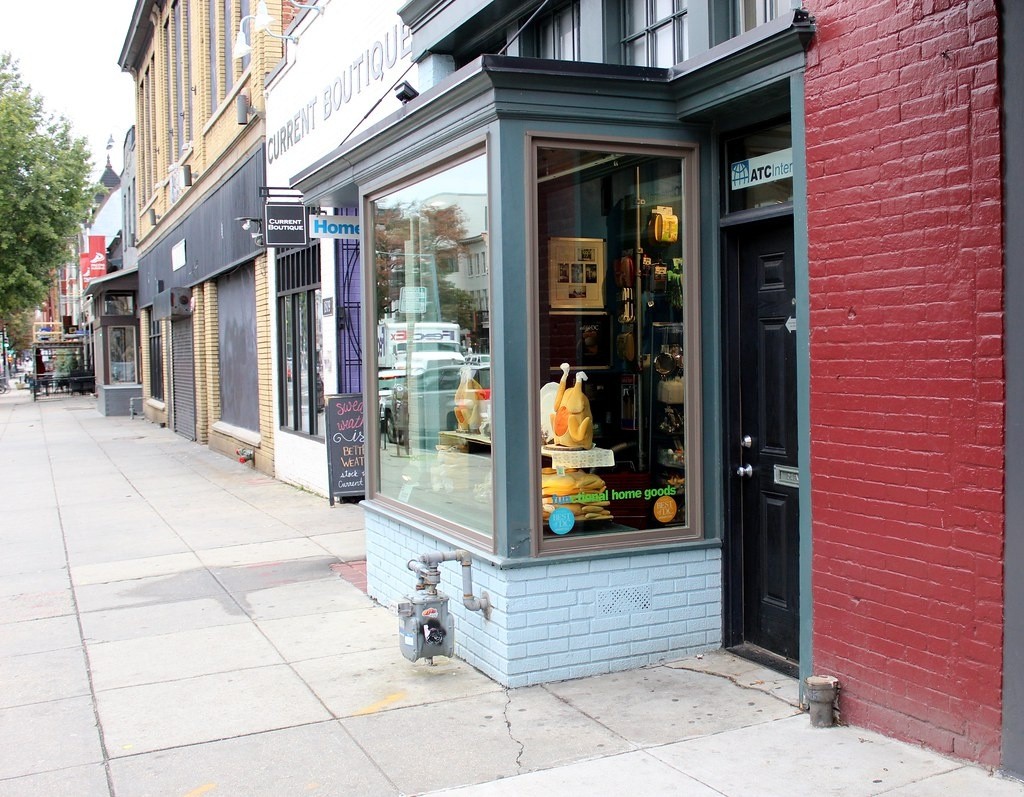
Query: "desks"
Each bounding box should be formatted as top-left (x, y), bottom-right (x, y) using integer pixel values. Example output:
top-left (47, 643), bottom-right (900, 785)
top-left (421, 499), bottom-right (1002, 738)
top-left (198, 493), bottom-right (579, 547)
top-left (437, 429), bottom-right (617, 524)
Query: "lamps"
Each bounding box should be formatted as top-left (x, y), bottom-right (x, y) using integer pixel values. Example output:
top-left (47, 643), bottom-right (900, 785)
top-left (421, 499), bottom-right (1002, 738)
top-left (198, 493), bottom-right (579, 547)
top-left (233, 15), bottom-right (299, 61)
top-left (237, 94), bottom-right (255, 125)
top-left (254, 0), bottom-right (324, 33)
top-left (394, 79), bottom-right (419, 105)
top-left (129, 234), bottom-right (139, 246)
top-left (149, 208), bottom-right (162, 225)
top-left (184, 164), bottom-right (199, 187)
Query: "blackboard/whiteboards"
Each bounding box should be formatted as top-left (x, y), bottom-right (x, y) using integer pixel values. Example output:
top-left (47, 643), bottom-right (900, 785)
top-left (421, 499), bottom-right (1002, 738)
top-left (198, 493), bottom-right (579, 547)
top-left (324, 393), bottom-right (365, 496)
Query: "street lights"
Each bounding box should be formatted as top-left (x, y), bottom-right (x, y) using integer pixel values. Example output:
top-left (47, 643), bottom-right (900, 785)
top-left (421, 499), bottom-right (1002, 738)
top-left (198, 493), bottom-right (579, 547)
top-left (13, 352), bottom-right (16, 369)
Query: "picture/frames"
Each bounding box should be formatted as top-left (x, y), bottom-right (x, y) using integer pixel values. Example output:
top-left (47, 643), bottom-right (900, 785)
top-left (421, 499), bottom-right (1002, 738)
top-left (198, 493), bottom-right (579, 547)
top-left (548, 235), bottom-right (605, 317)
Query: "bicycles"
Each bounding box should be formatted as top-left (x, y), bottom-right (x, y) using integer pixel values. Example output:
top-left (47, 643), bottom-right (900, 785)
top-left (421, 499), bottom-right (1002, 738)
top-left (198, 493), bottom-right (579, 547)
top-left (0, 383), bottom-right (11, 394)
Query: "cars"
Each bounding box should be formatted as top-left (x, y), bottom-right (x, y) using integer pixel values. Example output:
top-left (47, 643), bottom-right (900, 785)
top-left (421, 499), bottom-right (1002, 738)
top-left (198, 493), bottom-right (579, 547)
top-left (384, 365), bottom-right (490, 444)
top-left (377, 367), bottom-right (425, 420)
top-left (464, 354), bottom-right (490, 366)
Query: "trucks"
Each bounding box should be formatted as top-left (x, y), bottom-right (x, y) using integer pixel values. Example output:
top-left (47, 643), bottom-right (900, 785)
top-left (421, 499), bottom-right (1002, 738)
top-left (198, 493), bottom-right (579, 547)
top-left (377, 323), bottom-right (466, 369)
